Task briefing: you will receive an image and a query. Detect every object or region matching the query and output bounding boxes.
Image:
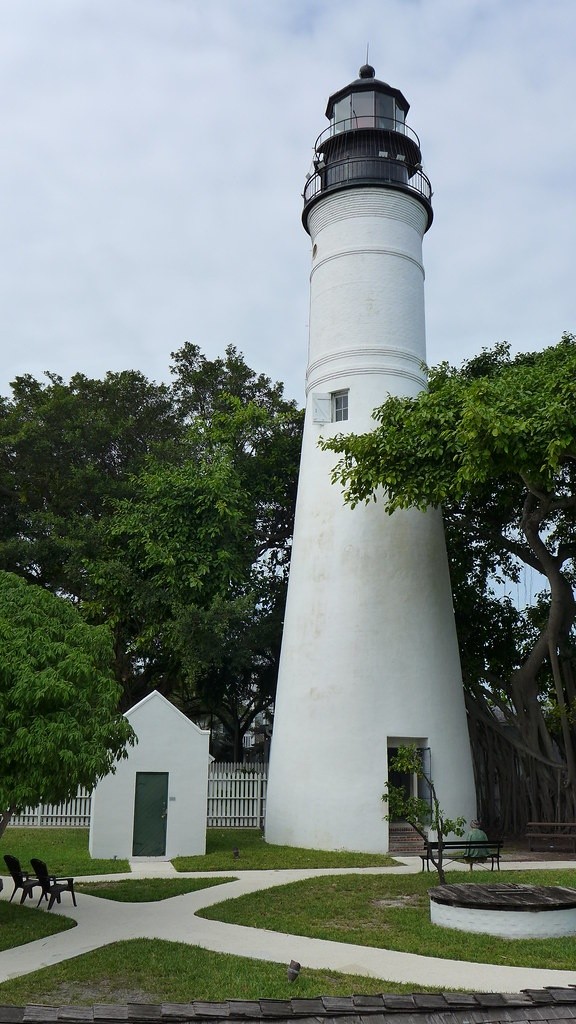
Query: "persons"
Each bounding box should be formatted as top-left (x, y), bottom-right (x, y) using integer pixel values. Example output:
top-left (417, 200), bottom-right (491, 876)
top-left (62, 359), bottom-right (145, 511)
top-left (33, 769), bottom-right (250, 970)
top-left (464, 820), bottom-right (488, 870)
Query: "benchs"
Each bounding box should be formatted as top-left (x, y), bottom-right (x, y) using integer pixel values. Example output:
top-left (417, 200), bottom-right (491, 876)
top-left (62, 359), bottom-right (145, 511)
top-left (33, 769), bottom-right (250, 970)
top-left (418, 838), bottom-right (504, 872)
top-left (524, 822), bottom-right (576, 852)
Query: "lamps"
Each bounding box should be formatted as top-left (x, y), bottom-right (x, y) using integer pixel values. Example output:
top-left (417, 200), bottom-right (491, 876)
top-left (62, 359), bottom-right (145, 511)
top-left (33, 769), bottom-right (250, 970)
top-left (233, 847), bottom-right (239, 857)
top-left (288, 959), bottom-right (300, 982)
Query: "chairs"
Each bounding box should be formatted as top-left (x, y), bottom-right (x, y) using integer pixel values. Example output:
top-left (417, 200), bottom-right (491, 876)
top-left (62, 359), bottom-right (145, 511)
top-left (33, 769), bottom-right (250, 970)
top-left (30, 858), bottom-right (77, 910)
top-left (3, 854), bottom-right (48, 905)
